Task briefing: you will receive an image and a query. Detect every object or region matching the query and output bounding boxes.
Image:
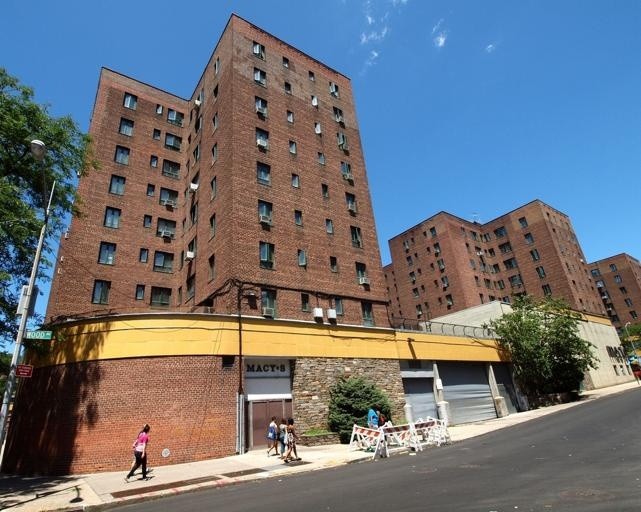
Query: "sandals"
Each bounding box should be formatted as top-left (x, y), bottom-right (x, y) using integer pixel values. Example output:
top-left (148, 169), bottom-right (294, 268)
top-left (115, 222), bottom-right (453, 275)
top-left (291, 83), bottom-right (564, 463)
top-left (123, 471), bottom-right (149, 483)
top-left (281, 456), bottom-right (302, 463)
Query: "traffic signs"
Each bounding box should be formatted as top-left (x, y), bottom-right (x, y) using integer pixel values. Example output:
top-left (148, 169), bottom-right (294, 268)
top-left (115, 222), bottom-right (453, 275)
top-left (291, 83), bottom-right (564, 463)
top-left (22, 330), bottom-right (52, 340)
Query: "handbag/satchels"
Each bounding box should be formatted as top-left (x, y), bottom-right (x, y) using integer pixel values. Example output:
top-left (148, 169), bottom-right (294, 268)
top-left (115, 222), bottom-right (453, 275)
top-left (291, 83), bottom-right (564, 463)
top-left (266, 427), bottom-right (280, 440)
top-left (284, 432), bottom-right (288, 444)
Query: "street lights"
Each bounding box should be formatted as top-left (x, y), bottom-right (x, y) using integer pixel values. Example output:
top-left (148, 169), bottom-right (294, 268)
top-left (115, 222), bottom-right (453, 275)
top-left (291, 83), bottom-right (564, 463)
top-left (624, 321), bottom-right (639, 363)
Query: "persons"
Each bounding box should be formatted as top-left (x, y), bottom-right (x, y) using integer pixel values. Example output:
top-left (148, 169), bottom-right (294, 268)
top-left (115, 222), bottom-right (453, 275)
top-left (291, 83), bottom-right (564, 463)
top-left (279, 418), bottom-right (293, 459)
top-left (368, 402), bottom-right (382, 428)
top-left (122, 423), bottom-right (151, 481)
top-left (283, 416), bottom-right (302, 463)
top-left (266, 416), bottom-right (280, 457)
top-left (132, 424), bottom-right (152, 474)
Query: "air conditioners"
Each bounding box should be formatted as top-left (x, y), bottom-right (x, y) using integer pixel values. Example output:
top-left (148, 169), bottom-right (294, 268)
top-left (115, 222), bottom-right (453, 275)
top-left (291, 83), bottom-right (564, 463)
top-left (184, 251), bottom-right (195, 262)
top-left (160, 230), bottom-right (172, 239)
top-left (188, 182), bottom-right (198, 193)
top-left (256, 139), bottom-right (266, 148)
top-left (261, 307), bottom-right (276, 317)
top-left (360, 277), bottom-right (371, 285)
top-left (192, 100), bottom-right (201, 110)
top-left (163, 200), bottom-right (174, 206)
